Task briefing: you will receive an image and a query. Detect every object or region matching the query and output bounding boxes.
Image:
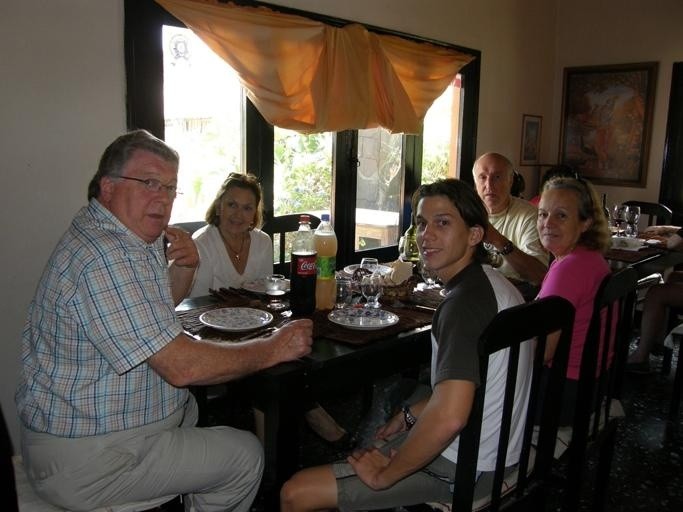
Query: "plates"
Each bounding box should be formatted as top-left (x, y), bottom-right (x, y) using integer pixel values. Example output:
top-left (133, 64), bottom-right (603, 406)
top-left (343, 263), bottom-right (393, 276)
top-left (240, 276), bottom-right (291, 294)
top-left (327, 307), bottom-right (400, 330)
top-left (198, 306), bottom-right (274, 331)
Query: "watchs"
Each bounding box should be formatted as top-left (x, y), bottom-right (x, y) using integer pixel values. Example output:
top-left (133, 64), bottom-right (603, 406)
top-left (498, 241), bottom-right (514, 255)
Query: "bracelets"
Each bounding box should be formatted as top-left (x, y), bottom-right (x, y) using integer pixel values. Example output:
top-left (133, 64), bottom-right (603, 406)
top-left (401, 404), bottom-right (417, 434)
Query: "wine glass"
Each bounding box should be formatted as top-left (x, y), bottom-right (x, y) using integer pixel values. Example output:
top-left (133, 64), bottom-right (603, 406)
top-left (361, 274), bottom-right (383, 309)
top-left (613, 203), bottom-right (640, 238)
top-left (265, 274), bottom-right (288, 308)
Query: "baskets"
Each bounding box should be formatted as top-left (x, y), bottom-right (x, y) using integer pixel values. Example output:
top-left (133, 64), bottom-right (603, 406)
top-left (352, 267), bottom-right (421, 302)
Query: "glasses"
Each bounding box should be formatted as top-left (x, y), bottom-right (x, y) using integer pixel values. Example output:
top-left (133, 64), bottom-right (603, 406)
top-left (119, 176), bottom-right (177, 201)
top-left (227, 172), bottom-right (240, 178)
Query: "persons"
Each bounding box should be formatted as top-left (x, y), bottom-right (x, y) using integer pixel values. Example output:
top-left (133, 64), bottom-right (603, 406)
top-left (526, 173), bottom-right (631, 431)
top-left (9, 126), bottom-right (316, 512)
top-left (180, 173), bottom-right (354, 479)
top-left (471, 151), bottom-right (549, 289)
top-left (277, 180), bottom-right (535, 512)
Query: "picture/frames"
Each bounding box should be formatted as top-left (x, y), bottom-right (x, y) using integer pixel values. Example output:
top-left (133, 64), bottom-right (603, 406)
top-left (519, 110), bottom-right (543, 168)
top-left (555, 57), bottom-right (659, 191)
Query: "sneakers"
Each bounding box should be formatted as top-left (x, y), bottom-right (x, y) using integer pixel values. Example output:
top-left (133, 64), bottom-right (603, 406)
top-left (635, 338), bottom-right (666, 356)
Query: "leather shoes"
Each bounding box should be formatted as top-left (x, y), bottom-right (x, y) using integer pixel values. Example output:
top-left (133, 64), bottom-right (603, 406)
top-left (622, 360), bottom-right (648, 370)
top-left (302, 414), bottom-right (358, 451)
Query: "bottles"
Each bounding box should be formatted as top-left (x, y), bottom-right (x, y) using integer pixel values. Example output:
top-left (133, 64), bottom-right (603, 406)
top-left (403, 209), bottom-right (419, 258)
top-left (599, 192), bottom-right (610, 223)
top-left (313, 214), bottom-right (338, 312)
top-left (288, 213), bottom-right (319, 316)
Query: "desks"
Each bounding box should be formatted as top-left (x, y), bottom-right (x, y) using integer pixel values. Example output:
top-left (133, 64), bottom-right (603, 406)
top-left (189, 228), bottom-right (680, 433)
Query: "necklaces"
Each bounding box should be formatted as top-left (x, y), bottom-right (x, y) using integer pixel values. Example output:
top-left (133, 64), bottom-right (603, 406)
top-left (217, 227), bottom-right (246, 260)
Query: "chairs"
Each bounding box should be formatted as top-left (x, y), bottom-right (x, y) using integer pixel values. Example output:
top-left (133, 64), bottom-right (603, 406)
top-left (523, 265), bottom-right (639, 511)
top-left (3, 405), bottom-right (186, 512)
top-left (610, 201), bottom-right (683, 454)
top-left (167, 213), bottom-right (320, 428)
top-left (379, 293), bottom-right (578, 512)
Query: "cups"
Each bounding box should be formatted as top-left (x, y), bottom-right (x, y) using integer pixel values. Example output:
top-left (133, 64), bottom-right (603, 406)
top-left (337, 276), bottom-right (362, 308)
top-left (360, 258), bottom-right (379, 274)
top-left (397, 236), bottom-right (418, 268)
